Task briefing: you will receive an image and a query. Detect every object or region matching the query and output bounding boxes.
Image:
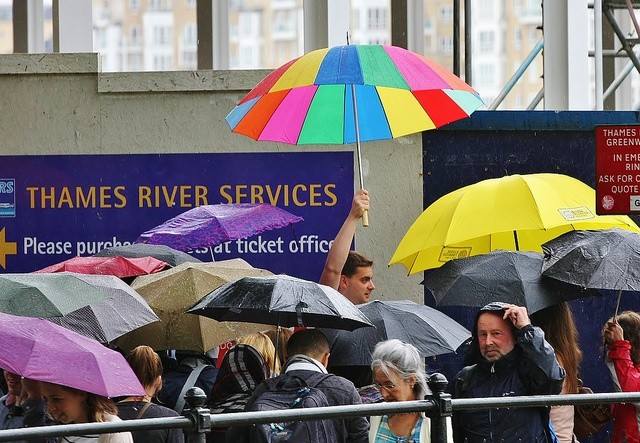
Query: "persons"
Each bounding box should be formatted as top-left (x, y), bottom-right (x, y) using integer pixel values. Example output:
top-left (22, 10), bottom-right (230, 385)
top-left (365, 340), bottom-right (456, 443)
top-left (445, 304), bottom-right (563, 443)
top-left (599, 313), bottom-right (640, 443)
top-left (310, 190), bottom-right (374, 301)
top-left (2, 375), bottom-right (130, 443)
top-left (527, 295), bottom-right (581, 439)
top-left (165, 328), bottom-right (293, 443)
top-left (122, 348), bottom-right (185, 443)
top-left (247, 328), bottom-right (371, 443)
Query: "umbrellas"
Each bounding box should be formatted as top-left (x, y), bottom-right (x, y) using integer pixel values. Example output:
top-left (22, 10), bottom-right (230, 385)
top-left (95, 243), bottom-right (203, 263)
top-left (307, 301), bottom-right (473, 367)
top-left (226, 32), bottom-right (487, 228)
top-left (32, 255), bottom-right (173, 278)
top-left (118, 263), bottom-right (290, 354)
top-left (541, 230), bottom-right (640, 356)
top-left (1, 273), bottom-right (162, 344)
top-left (388, 174), bottom-right (640, 273)
top-left (423, 252), bottom-right (602, 312)
top-left (0, 313), bottom-right (146, 399)
top-left (187, 276), bottom-right (377, 375)
top-left (133, 204), bottom-right (304, 248)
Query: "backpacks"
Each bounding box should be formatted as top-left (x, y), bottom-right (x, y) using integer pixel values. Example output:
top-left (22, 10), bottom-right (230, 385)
top-left (571, 377), bottom-right (609, 434)
top-left (254, 372), bottom-right (343, 442)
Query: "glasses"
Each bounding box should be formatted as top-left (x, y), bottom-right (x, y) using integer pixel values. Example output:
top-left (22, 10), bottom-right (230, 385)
top-left (373, 379), bottom-right (406, 392)
